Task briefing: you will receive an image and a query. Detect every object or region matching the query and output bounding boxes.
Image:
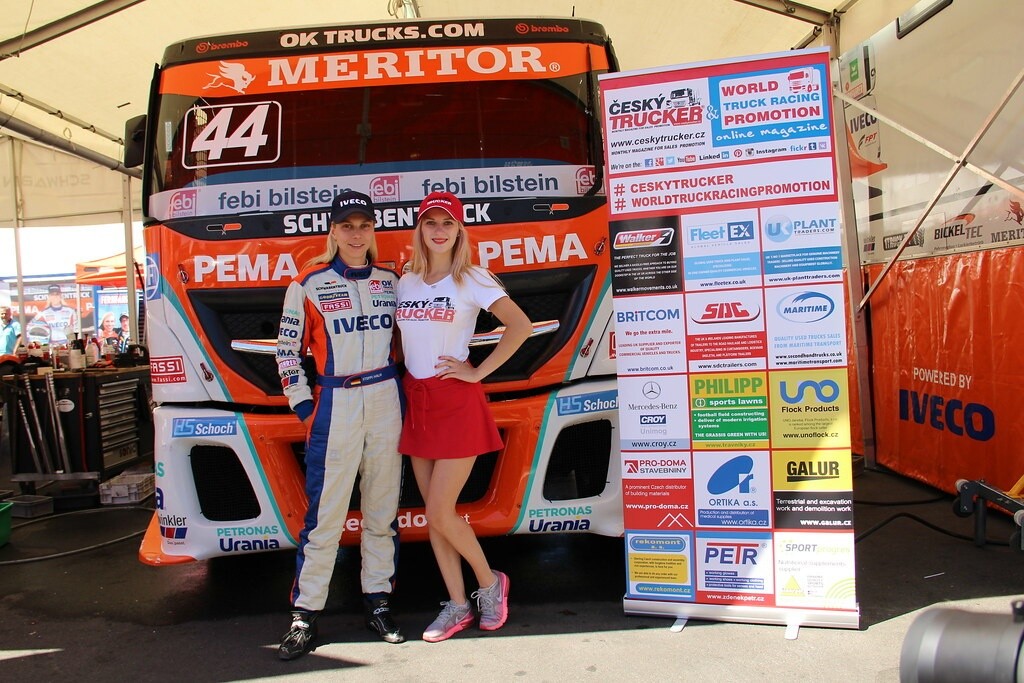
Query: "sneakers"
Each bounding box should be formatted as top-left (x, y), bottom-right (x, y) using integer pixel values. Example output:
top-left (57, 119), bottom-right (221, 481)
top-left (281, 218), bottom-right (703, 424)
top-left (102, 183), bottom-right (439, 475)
top-left (422, 600), bottom-right (474, 644)
top-left (279, 596), bottom-right (320, 659)
top-left (476, 569), bottom-right (509, 630)
top-left (364, 596), bottom-right (406, 644)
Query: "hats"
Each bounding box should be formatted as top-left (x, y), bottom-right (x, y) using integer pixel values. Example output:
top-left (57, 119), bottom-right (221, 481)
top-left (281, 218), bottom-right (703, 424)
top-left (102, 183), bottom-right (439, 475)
top-left (120, 311), bottom-right (129, 319)
top-left (417, 193), bottom-right (463, 224)
top-left (330, 192), bottom-right (376, 222)
top-left (49, 285), bottom-right (61, 293)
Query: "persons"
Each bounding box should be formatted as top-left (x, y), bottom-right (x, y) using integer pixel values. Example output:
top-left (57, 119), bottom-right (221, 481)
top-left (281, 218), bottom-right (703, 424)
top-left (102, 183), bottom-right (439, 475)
top-left (99, 312), bottom-right (119, 350)
top-left (26, 283), bottom-right (80, 351)
top-left (274, 190), bottom-right (409, 662)
top-left (114, 314), bottom-right (130, 353)
top-left (393, 190), bottom-right (534, 644)
top-left (0, 305), bottom-right (23, 356)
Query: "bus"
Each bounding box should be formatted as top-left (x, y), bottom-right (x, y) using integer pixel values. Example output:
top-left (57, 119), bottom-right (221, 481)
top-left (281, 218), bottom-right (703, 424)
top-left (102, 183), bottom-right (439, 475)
top-left (122, 17), bottom-right (624, 566)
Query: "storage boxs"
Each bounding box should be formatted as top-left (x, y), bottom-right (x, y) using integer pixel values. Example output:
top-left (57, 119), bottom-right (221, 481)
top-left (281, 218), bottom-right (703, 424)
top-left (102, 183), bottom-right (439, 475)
top-left (3, 494), bottom-right (55, 519)
top-left (0, 489), bottom-right (14, 503)
top-left (100, 473), bottom-right (155, 504)
top-left (0, 503), bottom-right (15, 547)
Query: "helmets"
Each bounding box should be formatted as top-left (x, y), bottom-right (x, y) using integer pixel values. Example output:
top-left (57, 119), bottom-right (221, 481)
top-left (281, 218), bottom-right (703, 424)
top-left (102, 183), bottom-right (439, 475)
top-left (27, 321), bottom-right (50, 344)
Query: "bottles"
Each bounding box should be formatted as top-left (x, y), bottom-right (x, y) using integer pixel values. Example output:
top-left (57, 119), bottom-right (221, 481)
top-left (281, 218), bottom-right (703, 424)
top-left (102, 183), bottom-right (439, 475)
top-left (69, 332), bottom-right (87, 372)
top-left (82, 334), bottom-right (101, 367)
